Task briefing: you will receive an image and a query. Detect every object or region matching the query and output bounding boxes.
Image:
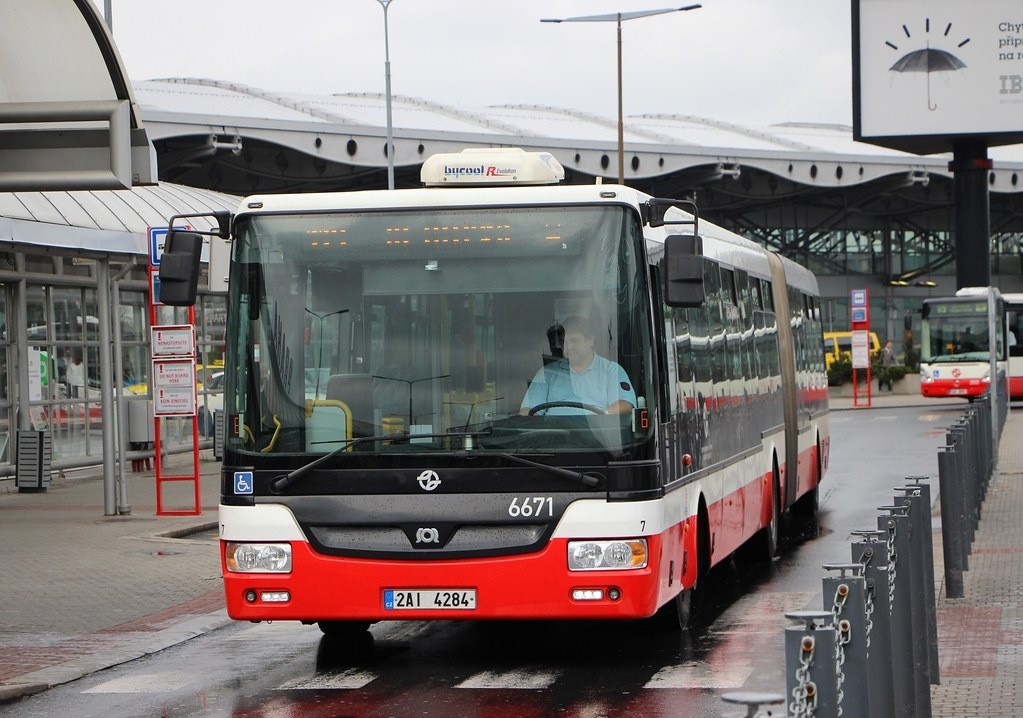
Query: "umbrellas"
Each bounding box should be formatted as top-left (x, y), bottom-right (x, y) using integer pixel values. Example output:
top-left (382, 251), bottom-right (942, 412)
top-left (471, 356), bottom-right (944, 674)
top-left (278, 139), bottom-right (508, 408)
top-left (890, 41), bottom-right (967, 110)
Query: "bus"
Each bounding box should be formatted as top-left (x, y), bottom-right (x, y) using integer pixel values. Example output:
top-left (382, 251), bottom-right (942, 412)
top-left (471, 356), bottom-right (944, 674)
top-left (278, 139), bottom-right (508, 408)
top-left (156, 147), bottom-right (831, 653)
top-left (28, 314), bottom-right (126, 425)
top-left (662, 305), bottom-right (831, 417)
top-left (902, 285), bottom-right (1023, 409)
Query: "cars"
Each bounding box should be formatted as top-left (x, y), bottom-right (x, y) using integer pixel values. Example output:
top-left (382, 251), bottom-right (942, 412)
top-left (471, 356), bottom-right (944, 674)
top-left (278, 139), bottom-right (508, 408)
top-left (126, 355), bottom-right (332, 438)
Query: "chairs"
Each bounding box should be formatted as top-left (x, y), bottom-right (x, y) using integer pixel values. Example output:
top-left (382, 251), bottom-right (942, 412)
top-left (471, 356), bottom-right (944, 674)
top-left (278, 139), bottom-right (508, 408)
top-left (327, 373), bottom-right (375, 450)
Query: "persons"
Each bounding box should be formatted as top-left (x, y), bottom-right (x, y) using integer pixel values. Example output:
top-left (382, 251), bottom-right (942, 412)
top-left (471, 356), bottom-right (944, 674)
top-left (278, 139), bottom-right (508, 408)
top-left (57, 347), bottom-right (86, 395)
top-left (955, 325), bottom-right (1022, 355)
top-left (519, 317), bottom-right (637, 421)
top-left (876, 341), bottom-right (900, 392)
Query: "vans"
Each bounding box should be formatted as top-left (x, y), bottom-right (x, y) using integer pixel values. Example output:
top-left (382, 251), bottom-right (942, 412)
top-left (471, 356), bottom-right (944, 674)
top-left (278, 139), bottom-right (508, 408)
top-left (821, 328), bottom-right (882, 381)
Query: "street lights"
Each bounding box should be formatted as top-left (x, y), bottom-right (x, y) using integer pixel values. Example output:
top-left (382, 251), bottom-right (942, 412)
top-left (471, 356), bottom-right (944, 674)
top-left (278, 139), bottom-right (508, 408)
top-left (538, 2), bottom-right (706, 186)
